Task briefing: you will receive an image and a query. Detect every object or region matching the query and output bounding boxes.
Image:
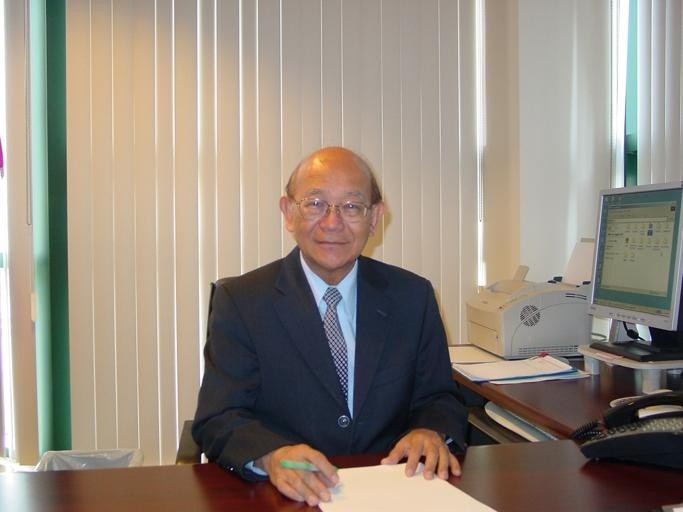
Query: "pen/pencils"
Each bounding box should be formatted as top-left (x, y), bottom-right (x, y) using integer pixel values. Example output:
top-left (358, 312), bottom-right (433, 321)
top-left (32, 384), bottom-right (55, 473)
top-left (279, 460), bottom-right (338, 472)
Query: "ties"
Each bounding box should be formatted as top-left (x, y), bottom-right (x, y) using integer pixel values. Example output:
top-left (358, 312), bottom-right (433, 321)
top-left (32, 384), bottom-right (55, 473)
top-left (322, 287), bottom-right (348, 402)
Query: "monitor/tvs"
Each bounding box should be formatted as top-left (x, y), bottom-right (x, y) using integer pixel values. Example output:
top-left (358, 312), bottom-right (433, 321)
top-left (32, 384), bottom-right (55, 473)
top-left (586, 181), bottom-right (683, 362)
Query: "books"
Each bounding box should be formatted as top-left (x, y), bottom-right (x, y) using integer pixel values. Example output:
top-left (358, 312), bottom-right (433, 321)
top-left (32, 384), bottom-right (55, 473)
top-left (307, 460), bottom-right (497, 511)
top-left (449, 353), bottom-right (578, 383)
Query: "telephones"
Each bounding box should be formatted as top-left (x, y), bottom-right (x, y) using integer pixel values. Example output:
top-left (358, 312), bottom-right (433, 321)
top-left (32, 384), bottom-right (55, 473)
top-left (579, 392), bottom-right (683, 470)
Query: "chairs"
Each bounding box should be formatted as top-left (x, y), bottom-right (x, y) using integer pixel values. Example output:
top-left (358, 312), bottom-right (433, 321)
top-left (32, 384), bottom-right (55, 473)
top-left (175, 278), bottom-right (237, 463)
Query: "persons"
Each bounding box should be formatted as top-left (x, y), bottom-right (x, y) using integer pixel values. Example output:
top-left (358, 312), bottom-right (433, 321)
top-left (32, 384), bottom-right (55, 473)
top-left (187, 146), bottom-right (471, 507)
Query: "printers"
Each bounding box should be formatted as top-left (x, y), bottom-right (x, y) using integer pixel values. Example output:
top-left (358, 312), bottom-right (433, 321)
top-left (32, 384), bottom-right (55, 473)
top-left (465, 237), bottom-right (594, 363)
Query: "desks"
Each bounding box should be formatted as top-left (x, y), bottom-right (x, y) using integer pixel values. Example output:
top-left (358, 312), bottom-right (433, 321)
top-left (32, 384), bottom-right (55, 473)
top-left (0, 344), bottom-right (683, 511)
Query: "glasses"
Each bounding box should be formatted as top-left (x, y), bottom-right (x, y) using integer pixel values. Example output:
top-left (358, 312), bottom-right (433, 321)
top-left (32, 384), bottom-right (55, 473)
top-left (289, 197), bottom-right (372, 218)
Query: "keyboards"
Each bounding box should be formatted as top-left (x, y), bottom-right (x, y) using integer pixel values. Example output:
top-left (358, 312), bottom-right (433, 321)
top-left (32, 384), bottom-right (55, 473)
top-left (483, 399), bottom-right (572, 443)
